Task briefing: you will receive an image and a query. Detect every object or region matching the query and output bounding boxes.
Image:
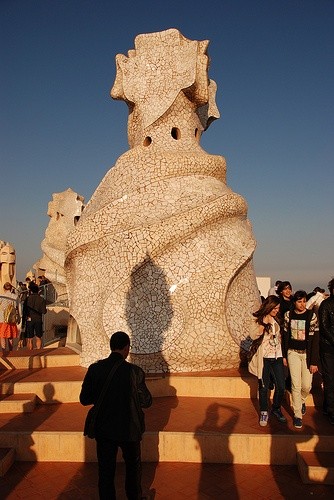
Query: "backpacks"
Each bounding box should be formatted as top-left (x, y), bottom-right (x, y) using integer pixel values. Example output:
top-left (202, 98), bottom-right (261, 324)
top-left (0, 302), bottom-right (17, 325)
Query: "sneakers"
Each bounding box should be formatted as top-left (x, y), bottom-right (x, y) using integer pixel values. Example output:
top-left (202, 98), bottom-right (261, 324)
top-left (271, 408), bottom-right (286, 423)
top-left (260, 411), bottom-right (269, 426)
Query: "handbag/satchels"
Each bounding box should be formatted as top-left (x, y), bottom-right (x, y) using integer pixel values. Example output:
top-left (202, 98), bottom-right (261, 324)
top-left (83, 405), bottom-right (96, 439)
top-left (239, 317), bottom-right (265, 362)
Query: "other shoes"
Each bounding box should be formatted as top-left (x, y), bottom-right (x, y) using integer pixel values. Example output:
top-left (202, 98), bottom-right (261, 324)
top-left (302, 403), bottom-right (306, 415)
top-left (327, 417), bottom-right (334, 425)
top-left (293, 418), bottom-right (303, 429)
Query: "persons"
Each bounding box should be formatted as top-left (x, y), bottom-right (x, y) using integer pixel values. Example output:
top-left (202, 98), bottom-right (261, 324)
top-left (22, 283), bottom-right (46, 350)
top-left (318, 280), bottom-right (334, 425)
top-left (276, 281), bottom-right (293, 327)
top-left (79, 331), bottom-right (152, 500)
top-left (307, 287), bottom-right (327, 313)
top-left (17, 276), bottom-right (50, 300)
top-left (281, 291), bottom-right (318, 429)
top-left (0, 282), bottom-right (19, 352)
top-left (269, 281), bottom-right (281, 297)
top-left (248, 295), bottom-right (287, 426)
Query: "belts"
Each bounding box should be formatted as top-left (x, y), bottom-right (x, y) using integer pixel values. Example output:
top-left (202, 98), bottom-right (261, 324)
top-left (288, 348), bottom-right (305, 354)
